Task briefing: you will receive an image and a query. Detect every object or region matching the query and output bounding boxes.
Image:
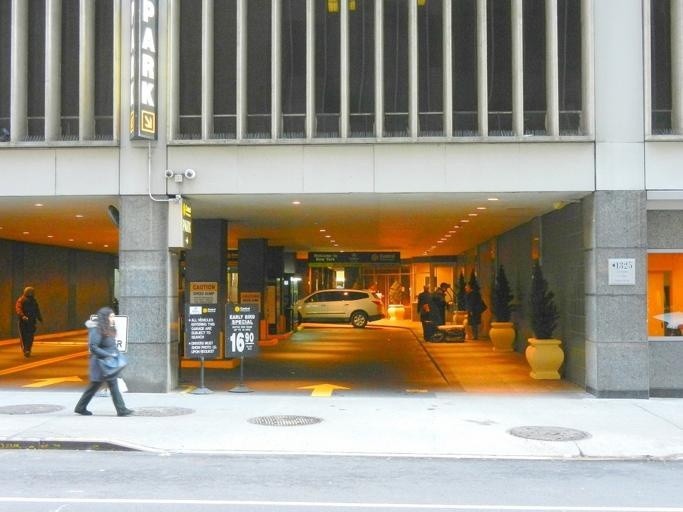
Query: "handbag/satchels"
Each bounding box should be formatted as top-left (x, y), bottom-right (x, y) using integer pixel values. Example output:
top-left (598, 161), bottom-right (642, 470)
top-left (97, 347), bottom-right (129, 381)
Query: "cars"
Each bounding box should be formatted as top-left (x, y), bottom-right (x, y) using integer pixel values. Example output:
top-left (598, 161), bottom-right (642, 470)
top-left (295, 288), bottom-right (387, 328)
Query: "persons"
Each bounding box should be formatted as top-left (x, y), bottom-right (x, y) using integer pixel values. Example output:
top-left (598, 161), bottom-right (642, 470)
top-left (15, 286), bottom-right (43, 358)
top-left (74, 306), bottom-right (135, 416)
top-left (427, 282), bottom-right (450, 324)
top-left (461, 283), bottom-right (488, 341)
top-left (422, 303), bottom-right (442, 339)
top-left (415, 284), bottom-right (430, 322)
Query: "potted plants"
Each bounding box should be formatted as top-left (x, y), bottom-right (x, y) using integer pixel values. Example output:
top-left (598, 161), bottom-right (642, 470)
top-left (525, 259), bottom-right (564, 380)
top-left (489, 262), bottom-right (517, 352)
top-left (388, 281), bottom-right (407, 320)
top-left (452, 266), bottom-right (488, 339)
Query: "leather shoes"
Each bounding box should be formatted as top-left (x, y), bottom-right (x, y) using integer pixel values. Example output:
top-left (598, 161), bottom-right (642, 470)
top-left (116, 407), bottom-right (135, 416)
top-left (73, 409), bottom-right (93, 416)
